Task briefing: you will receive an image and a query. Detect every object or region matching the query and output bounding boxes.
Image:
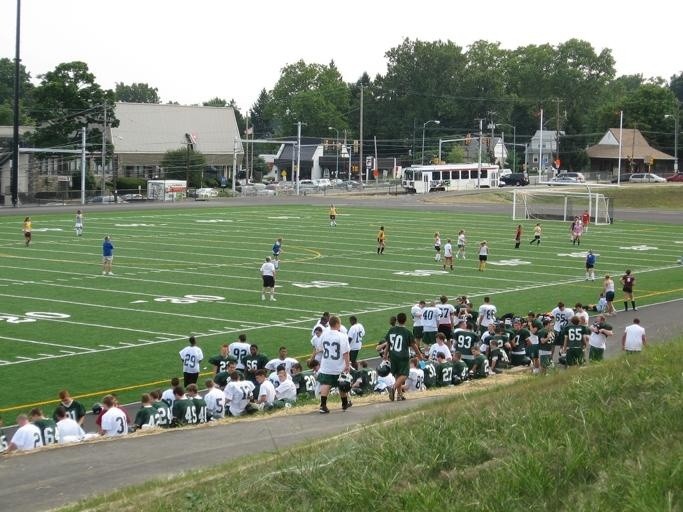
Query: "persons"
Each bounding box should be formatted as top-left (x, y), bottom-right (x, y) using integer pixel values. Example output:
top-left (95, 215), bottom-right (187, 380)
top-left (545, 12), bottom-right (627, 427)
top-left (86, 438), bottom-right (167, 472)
top-left (622, 318), bottom-right (647, 355)
top-left (477, 240), bottom-right (489, 272)
top-left (1, 390), bottom-right (87, 453)
top-left (585, 250), bottom-right (597, 282)
top-left (272, 238), bottom-right (283, 262)
top-left (74, 210), bottom-right (82, 236)
top-left (376, 295), bottom-right (614, 401)
top-left (306, 311), bottom-right (378, 413)
top-left (179, 334), bottom-right (299, 393)
top-left (569, 209), bottom-right (590, 245)
top-left (515, 223), bottom-right (522, 248)
top-left (259, 256), bottom-right (277, 302)
top-left (602, 275), bottom-right (617, 317)
top-left (102, 236), bottom-right (115, 276)
top-left (21, 217), bottom-right (31, 247)
top-left (96, 360), bottom-right (318, 434)
top-left (328, 204), bottom-right (339, 227)
top-left (529, 222), bottom-right (542, 246)
top-left (432, 229), bottom-right (466, 272)
top-left (619, 269), bottom-right (638, 311)
top-left (376, 225), bottom-right (385, 255)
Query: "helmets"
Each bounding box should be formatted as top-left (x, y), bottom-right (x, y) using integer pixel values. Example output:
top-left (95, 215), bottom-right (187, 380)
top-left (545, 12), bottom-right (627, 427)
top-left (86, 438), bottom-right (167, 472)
top-left (92, 403), bottom-right (104, 414)
top-left (452, 375), bottom-right (461, 384)
top-left (376, 360), bottom-right (391, 377)
top-left (337, 371), bottom-right (353, 392)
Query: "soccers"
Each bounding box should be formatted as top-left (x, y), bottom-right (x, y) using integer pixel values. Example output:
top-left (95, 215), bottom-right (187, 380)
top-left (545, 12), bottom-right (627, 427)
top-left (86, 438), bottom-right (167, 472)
top-left (675, 259), bottom-right (682, 264)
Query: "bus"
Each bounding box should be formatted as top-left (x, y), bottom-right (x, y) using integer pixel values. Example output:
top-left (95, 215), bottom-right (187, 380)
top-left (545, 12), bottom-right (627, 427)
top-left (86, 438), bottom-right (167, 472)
top-left (401, 161), bottom-right (500, 194)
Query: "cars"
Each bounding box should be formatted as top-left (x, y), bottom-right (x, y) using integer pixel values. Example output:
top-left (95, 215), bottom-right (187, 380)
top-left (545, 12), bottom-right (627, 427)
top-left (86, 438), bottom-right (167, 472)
top-left (501, 173), bottom-right (529, 186)
top-left (550, 172), bottom-right (584, 182)
top-left (88, 178), bottom-right (367, 203)
top-left (610, 171), bottom-right (682, 183)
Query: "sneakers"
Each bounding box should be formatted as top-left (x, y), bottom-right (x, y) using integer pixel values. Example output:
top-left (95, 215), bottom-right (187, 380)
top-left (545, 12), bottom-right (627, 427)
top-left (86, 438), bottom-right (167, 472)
top-left (397, 391), bottom-right (406, 401)
top-left (343, 400), bottom-right (352, 410)
top-left (262, 295), bottom-right (276, 302)
top-left (103, 271), bottom-right (113, 275)
top-left (385, 384), bottom-right (395, 401)
top-left (319, 406), bottom-right (329, 413)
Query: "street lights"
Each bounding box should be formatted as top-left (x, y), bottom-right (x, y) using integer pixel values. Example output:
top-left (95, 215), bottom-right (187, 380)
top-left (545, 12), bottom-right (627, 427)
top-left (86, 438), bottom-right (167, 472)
top-left (421, 120), bottom-right (440, 165)
top-left (328, 127), bottom-right (338, 178)
top-left (664, 114), bottom-right (678, 174)
top-left (496, 123), bottom-right (515, 173)
